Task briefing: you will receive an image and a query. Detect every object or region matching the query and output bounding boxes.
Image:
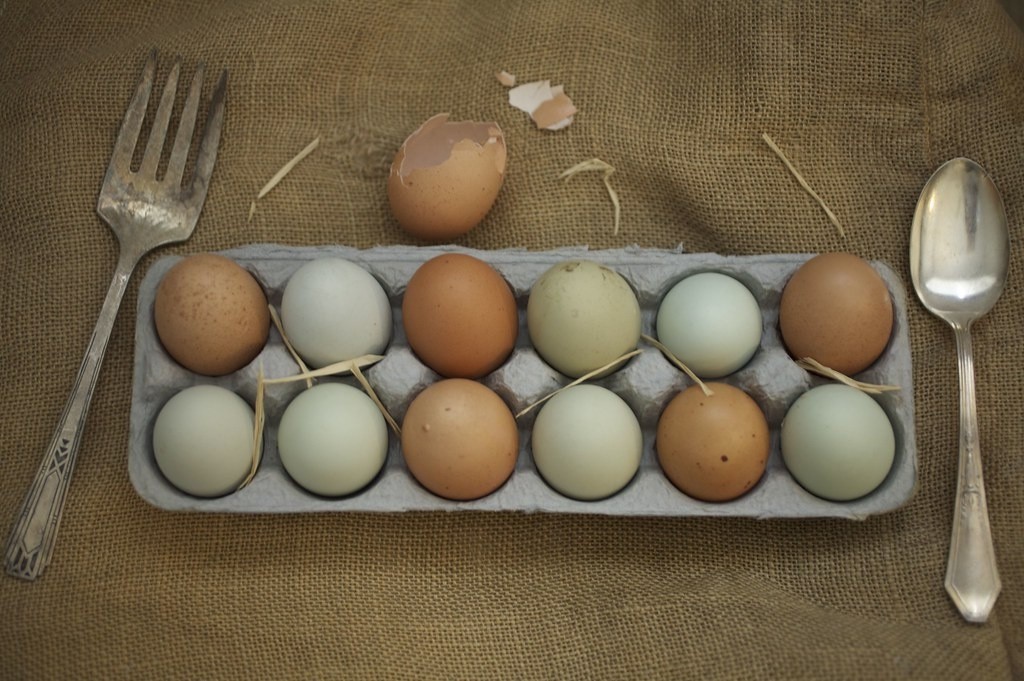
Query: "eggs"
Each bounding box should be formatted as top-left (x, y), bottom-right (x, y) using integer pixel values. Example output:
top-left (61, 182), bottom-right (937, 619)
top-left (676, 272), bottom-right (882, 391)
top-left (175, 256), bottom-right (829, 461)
top-left (151, 67), bottom-right (901, 503)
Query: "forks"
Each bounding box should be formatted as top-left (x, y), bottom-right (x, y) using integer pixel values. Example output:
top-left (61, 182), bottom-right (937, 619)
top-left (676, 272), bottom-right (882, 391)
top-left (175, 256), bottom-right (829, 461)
top-left (0, 44), bottom-right (226, 580)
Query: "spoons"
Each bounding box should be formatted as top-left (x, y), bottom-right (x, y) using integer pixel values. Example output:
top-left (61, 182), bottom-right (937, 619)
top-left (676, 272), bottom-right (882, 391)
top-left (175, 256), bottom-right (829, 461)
top-left (908, 155), bottom-right (1009, 624)
